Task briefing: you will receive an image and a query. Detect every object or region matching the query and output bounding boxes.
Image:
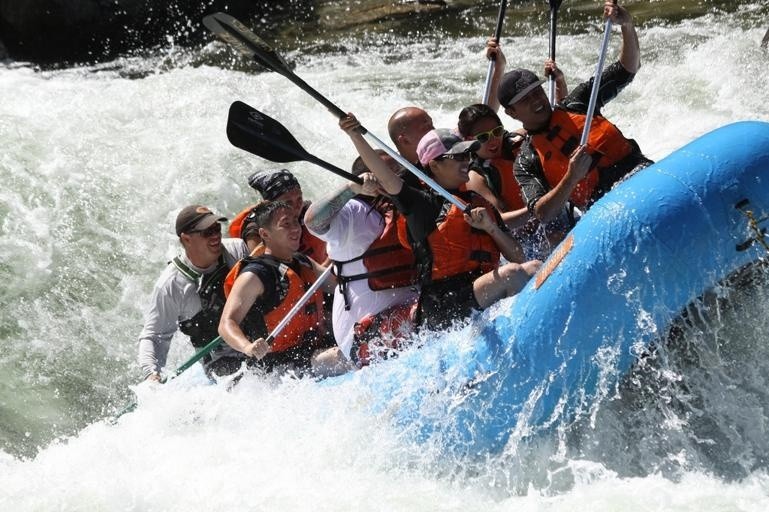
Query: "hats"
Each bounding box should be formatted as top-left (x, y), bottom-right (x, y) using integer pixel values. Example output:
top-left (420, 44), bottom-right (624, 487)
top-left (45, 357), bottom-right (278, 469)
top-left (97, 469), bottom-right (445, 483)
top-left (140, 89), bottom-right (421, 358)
top-left (248, 168), bottom-right (300, 200)
top-left (176, 205), bottom-right (228, 236)
top-left (498, 68), bottom-right (546, 107)
top-left (416, 128), bottom-right (481, 168)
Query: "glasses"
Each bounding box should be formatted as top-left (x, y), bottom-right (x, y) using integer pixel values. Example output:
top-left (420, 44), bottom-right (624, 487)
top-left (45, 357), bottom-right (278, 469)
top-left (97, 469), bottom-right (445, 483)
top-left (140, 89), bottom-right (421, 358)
top-left (442, 150), bottom-right (471, 161)
top-left (467, 125), bottom-right (504, 145)
top-left (191, 223), bottom-right (221, 238)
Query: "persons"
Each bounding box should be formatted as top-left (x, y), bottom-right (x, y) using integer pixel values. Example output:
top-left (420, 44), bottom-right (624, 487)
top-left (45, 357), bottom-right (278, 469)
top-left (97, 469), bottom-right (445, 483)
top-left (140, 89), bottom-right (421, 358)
top-left (138, 38), bottom-right (582, 390)
top-left (496, 0), bottom-right (655, 223)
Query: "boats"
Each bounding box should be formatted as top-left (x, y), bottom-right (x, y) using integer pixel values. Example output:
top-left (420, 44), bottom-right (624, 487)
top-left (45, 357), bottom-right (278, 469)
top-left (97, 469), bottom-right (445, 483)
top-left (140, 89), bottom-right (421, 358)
top-left (314, 119), bottom-right (769, 464)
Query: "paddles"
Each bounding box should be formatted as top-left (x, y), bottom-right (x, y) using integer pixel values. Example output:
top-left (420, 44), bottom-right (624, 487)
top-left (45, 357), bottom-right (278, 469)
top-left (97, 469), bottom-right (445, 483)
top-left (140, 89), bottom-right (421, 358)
top-left (203, 12), bottom-right (473, 218)
top-left (226, 101), bottom-right (393, 197)
